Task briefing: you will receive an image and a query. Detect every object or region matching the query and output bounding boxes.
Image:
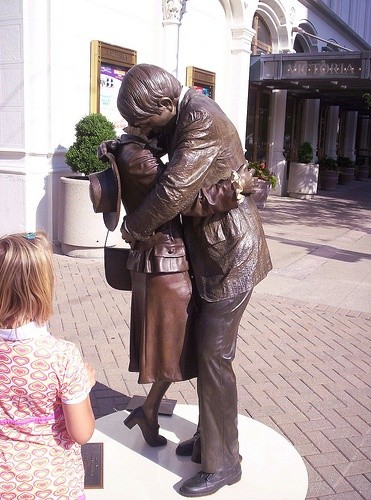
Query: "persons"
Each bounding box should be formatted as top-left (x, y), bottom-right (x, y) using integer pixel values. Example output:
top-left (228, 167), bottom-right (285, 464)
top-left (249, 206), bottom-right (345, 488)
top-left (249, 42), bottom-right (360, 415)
top-left (0, 231), bottom-right (96, 500)
top-left (91, 121), bottom-right (259, 447)
top-left (117, 64), bottom-right (273, 498)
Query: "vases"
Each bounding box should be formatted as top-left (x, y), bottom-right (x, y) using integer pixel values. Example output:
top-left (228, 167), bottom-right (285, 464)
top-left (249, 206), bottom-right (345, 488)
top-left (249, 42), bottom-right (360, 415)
top-left (251, 179), bottom-right (270, 209)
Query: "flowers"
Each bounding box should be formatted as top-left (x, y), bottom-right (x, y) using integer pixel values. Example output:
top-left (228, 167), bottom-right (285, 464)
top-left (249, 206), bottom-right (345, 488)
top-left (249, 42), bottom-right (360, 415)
top-left (247, 160), bottom-right (278, 191)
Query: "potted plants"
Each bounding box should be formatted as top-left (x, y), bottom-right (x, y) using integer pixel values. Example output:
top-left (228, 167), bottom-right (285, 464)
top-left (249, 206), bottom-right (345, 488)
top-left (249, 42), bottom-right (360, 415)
top-left (58, 113), bottom-right (118, 260)
top-left (288, 141), bottom-right (319, 199)
top-left (338, 157), bottom-right (355, 185)
top-left (318, 157), bottom-right (340, 191)
top-left (355, 155), bottom-right (370, 180)
